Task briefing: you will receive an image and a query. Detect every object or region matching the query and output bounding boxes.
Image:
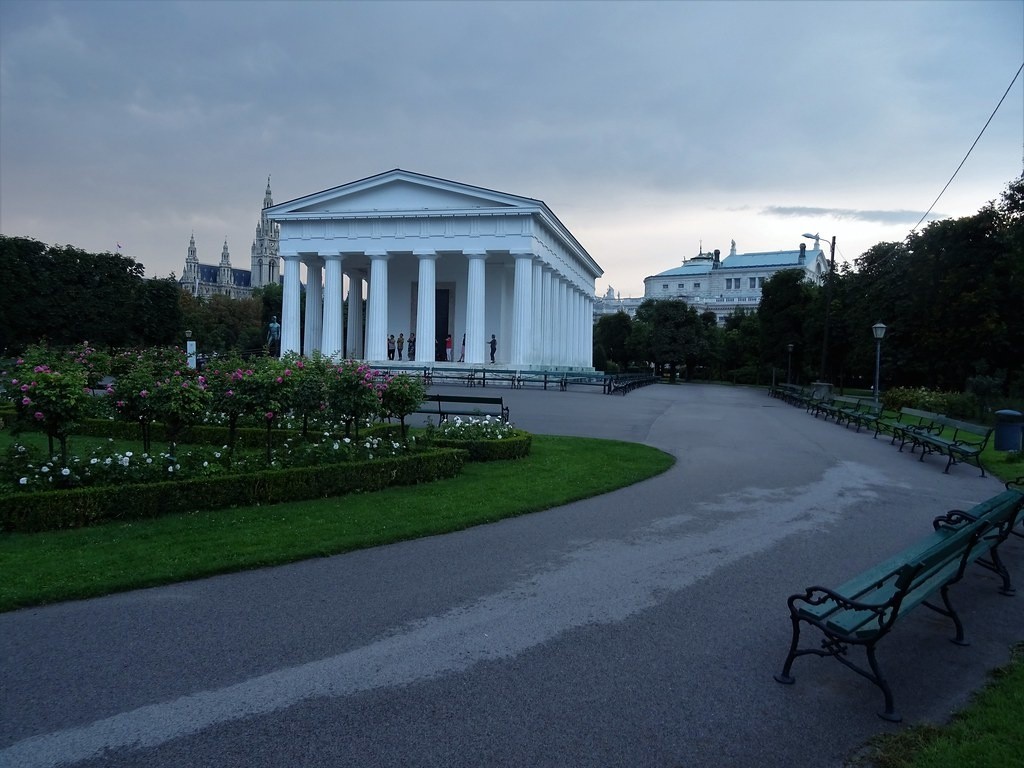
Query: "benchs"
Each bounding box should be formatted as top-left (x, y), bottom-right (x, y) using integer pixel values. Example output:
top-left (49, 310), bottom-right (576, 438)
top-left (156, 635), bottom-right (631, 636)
top-left (805, 391), bottom-right (826, 415)
top-left (815, 396), bottom-right (848, 420)
top-left (907, 415), bottom-right (994, 478)
top-left (370, 366), bottom-right (388, 385)
top-left (932, 476), bottom-right (1024, 591)
top-left (611, 373), bottom-right (661, 396)
top-left (430, 367), bottom-right (473, 387)
top-left (564, 372), bottom-right (612, 394)
top-left (768, 382), bottom-right (814, 407)
top-left (471, 368), bottom-right (516, 388)
top-left (517, 369), bottom-right (564, 392)
top-left (842, 400), bottom-right (885, 435)
top-left (407, 393), bottom-right (510, 424)
top-left (388, 365), bottom-right (431, 386)
top-left (825, 398), bottom-right (860, 425)
top-left (773, 502), bottom-right (1012, 721)
top-left (873, 407), bottom-right (947, 453)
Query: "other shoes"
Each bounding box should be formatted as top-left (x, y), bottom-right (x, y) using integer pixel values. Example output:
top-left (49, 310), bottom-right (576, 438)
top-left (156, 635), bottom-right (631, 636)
top-left (409, 359), bottom-right (414, 361)
top-left (462, 361), bottom-right (464, 362)
top-left (398, 357), bottom-right (402, 361)
top-left (491, 360), bottom-right (494, 363)
top-left (457, 359), bottom-right (461, 362)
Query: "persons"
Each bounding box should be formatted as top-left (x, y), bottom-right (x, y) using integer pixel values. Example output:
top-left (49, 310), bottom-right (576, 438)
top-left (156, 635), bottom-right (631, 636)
top-left (266, 315), bottom-right (280, 358)
top-left (387, 332), bottom-right (439, 361)
top-left (457, 333), bottom-right (466, 362)
top-left (445, 334), bottom-right (453, 361)
top-left (487, 334), bottom-right (497, 363)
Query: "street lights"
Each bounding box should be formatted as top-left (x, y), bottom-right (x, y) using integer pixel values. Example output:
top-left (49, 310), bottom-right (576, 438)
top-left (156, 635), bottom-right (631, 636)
top-left (802, 233), bottom-right (836, 382)
top-left (786, 343), bottom-right (794, 384)
top-left (871, 320), bottom-right (887, 414)
